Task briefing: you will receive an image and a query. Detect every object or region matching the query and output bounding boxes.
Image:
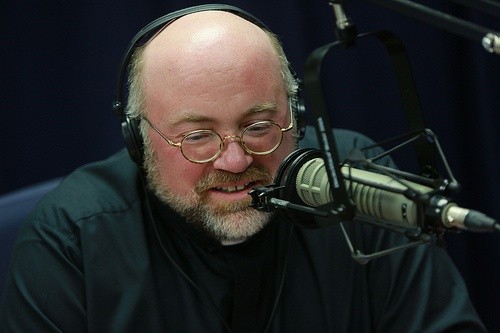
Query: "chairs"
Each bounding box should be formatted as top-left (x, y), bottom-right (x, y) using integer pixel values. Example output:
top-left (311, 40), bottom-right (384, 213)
top-left (0, 175), bottom-right (65, 288)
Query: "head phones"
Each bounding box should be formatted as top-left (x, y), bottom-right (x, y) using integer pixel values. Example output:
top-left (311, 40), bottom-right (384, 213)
top-left (113, 4), bottom-right (308, 166)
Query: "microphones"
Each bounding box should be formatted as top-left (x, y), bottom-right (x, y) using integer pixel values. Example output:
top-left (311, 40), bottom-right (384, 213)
top-left (296, 157), bottom-right (500, 233)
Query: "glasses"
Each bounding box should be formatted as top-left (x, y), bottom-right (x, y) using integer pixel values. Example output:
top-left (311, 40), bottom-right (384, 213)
top-left (139, 98), bottom-right (294, 164)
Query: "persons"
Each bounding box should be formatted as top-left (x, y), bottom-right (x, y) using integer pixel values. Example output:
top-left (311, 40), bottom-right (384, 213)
top-left (0, 10), bottom-right (486, 333)
